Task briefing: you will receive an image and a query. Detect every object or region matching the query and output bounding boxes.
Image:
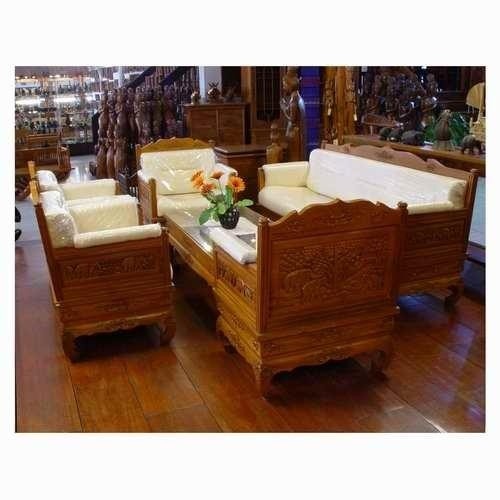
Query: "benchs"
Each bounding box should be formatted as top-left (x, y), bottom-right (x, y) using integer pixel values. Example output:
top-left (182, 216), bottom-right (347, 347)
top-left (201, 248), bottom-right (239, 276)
top-left (16, 133), bottom-right (70, 180)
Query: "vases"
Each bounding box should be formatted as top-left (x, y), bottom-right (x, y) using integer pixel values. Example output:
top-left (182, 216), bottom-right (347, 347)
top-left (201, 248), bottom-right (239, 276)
top-left (218, 210), bottom-right (239, 230)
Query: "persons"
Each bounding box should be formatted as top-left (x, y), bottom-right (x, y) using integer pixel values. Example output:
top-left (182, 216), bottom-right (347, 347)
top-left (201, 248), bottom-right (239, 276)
top-left (359, 73), bottom-right (447, 146)
top-left (264, 124), bottom-right (283, 165)
top-left (277, 66), bottom-right (306, 162)
top-left (90, 82), bottom-right (190, 178)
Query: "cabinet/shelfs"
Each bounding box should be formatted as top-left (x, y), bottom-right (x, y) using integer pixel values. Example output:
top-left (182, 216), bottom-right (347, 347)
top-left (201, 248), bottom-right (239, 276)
top-left (182, 102), bottom-right (247, 146)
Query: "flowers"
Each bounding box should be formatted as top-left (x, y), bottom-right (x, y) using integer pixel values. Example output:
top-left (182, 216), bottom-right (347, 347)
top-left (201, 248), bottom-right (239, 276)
top-left (188, 169), bottom-right (255, 225)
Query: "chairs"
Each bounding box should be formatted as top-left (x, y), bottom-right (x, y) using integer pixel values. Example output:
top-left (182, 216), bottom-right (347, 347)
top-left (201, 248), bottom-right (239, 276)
top-left (208, 198), bottom-right (409, 402)
top-left (27, 159), bottom-right (132, 205)
top-left (134, 136), bottom-right (240, 225)
top-left (27, 180), bottom-right (176, 360)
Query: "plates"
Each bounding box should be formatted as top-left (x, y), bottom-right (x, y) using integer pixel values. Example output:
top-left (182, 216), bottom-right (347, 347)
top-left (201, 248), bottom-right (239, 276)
top-left (200, 216), bottom-right (257, 235)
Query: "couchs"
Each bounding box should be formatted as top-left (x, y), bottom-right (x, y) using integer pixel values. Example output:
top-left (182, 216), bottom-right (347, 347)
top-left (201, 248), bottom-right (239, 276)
top-left (257, 139), bottom-right (479, 312)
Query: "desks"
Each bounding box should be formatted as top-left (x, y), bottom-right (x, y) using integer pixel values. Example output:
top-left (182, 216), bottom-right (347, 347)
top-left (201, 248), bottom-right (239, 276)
top-left (214, 144), bottom-right (268, 175)
top-left (339, 132), bottom-right (484, 270)
